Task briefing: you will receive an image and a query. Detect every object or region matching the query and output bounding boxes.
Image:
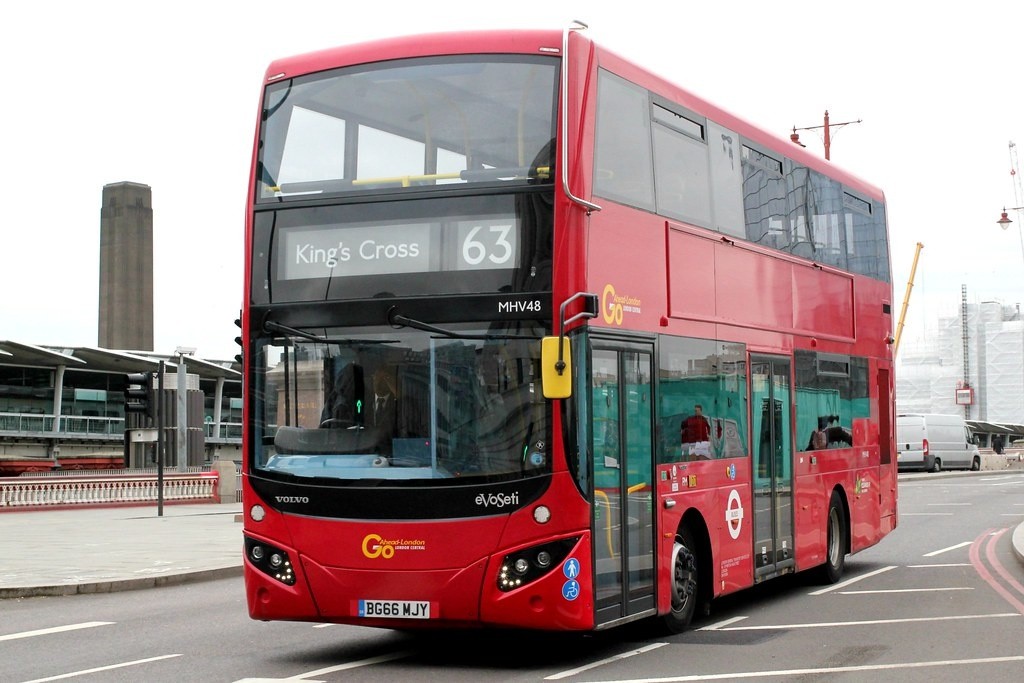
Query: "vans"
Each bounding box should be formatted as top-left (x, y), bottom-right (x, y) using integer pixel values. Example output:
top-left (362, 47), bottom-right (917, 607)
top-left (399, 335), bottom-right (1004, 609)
top-left (895, 412), bottom-right (984, 473)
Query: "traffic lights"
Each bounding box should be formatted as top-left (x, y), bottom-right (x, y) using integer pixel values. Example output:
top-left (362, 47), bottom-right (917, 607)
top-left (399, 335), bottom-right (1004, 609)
top-left (234, 318), bottom-right (243, 364)
top-left (122, 370), bottom-right (154, 417)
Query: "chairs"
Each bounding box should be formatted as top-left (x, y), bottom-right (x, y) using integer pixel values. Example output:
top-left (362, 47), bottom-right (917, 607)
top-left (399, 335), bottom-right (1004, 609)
top-left (756, 225), bottom-right (844, 270)
top-left (319, 395), bottom-right (361, 429)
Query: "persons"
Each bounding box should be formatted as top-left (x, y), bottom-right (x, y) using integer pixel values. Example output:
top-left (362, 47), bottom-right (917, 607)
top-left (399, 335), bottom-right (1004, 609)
top-left (680, 403), bottom-right (715, 460)
top-left (351, 369), bottom-right (411, 449)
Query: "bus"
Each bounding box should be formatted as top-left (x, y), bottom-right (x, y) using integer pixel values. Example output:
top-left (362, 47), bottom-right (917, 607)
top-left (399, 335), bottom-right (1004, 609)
top-left (241, 28), bottom-right (900, 640)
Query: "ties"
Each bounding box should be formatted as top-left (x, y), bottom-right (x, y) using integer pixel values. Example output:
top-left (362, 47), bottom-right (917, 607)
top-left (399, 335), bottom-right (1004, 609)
top-left (375, 398), bottom-right (385, 426)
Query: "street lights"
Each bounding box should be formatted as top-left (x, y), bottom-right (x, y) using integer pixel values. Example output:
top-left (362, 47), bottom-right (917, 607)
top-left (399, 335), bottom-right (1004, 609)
top-left (996, 137), bottom-right (1024, 267)
